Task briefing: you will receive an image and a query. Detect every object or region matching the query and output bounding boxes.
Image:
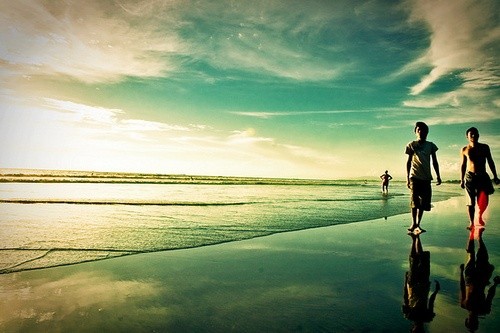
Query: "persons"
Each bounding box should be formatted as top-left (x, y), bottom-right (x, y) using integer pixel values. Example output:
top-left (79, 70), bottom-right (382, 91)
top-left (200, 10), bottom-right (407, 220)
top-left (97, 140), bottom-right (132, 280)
top-left (401, 231), bottom-right (439, 333)
top-left (460, 126), bottom-right (500, 229)
top-left (380, 170), bottom-right (393, 192)
top-left (405, 121), bottom-right (442, 232)
top-left (460, 227), bottom-right (500, 330)
